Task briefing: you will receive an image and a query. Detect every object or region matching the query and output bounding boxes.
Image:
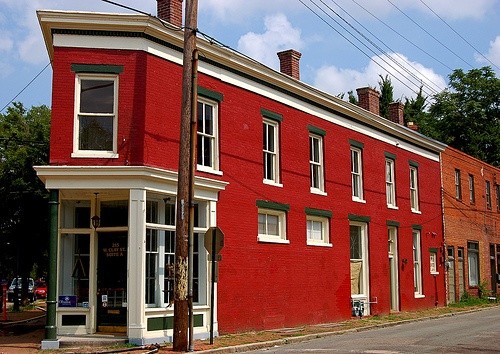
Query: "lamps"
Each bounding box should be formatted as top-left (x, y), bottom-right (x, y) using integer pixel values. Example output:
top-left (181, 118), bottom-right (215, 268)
top-left (91, 193), bottom-right (102, 230)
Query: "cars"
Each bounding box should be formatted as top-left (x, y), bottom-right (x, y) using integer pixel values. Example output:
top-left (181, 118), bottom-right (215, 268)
top-left (8, 277), bottom-right (47, 302)
